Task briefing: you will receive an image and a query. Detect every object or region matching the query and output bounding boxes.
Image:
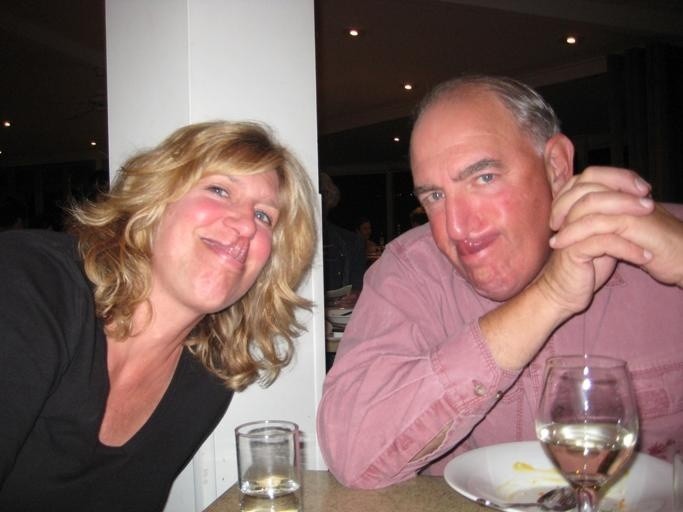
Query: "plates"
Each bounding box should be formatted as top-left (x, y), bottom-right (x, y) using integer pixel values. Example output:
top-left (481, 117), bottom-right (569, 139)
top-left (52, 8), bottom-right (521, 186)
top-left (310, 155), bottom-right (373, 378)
top-left (326, 307), bottom-right (352, 325)
top-left (442, 441), bottom-right (675, 512)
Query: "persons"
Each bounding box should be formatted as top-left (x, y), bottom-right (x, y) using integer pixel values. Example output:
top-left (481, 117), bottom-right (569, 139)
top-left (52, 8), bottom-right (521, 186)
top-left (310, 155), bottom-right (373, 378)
top-left (354, 214), bottom-right (379, 251)
top-left (383, 231), bottom-right (399, 247)
top-left (312, 69), bottom-right (683, 493)
top-left (410, 204), bottom-right (430, 228)
top-left (0, 118), bottom-right (321, 510)
top-left (318, 172), bottom-right (371, 309)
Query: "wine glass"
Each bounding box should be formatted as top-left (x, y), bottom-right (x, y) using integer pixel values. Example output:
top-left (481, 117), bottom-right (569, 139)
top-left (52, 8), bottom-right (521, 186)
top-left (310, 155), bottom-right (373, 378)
top-left (535, 353), bottom-right (639, 512)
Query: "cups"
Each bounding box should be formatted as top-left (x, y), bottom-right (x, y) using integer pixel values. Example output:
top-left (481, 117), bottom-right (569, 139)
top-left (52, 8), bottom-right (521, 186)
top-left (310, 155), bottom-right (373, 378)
top-left (234, 420), bottom-right (301, 512)
